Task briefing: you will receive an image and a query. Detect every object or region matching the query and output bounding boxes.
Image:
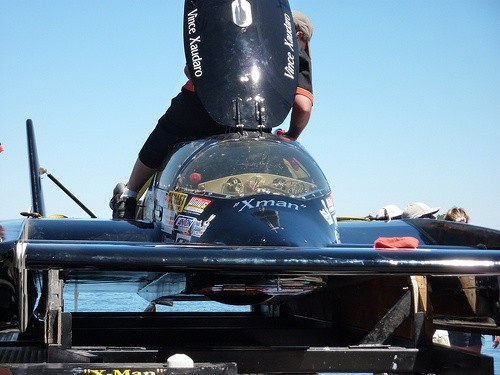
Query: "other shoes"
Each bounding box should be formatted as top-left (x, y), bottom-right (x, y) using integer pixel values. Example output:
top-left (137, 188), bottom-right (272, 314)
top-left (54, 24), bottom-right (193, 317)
top-left (109, 182), bottom-right (137, 219)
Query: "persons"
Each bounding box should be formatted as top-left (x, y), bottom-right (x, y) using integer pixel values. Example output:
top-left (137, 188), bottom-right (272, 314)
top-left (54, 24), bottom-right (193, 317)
top-left (112, 8), bottom-right (312, 219)
top-left (376, 202), bottom-right (468, 224)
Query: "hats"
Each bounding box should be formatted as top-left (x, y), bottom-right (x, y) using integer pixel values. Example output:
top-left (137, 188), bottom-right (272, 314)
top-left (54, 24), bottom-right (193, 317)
top-left (401, 203), bottom-right (440, 219)
